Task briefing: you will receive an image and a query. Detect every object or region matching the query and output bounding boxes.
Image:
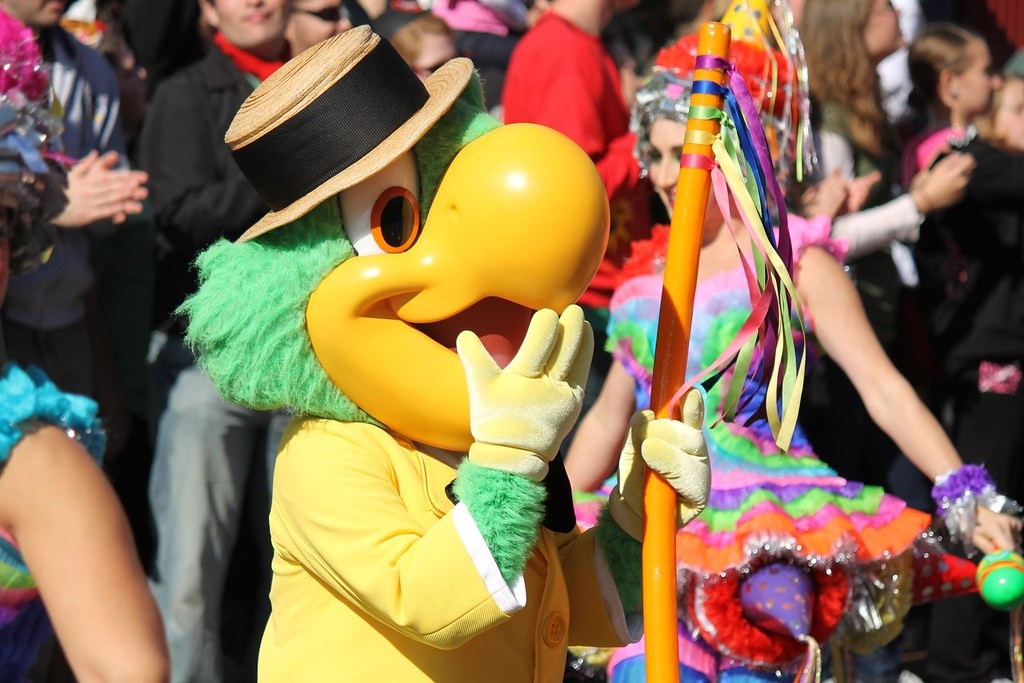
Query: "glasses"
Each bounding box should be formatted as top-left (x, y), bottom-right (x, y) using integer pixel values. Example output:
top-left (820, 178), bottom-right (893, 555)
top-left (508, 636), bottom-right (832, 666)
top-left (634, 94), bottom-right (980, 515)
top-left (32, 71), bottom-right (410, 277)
top-left (412, 46), bottom-right (464, 74)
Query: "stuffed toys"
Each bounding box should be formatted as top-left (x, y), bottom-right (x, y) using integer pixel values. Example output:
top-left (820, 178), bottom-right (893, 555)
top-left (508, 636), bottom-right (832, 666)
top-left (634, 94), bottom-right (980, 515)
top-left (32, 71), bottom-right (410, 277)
top-left (176, 24), bottom-right (711, 683)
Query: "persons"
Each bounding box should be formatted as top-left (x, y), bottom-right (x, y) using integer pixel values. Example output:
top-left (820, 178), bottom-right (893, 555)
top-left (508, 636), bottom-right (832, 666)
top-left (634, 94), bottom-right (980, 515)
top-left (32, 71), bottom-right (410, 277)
top-left (687, 0), bottom-right (1024, 513)
top-left (564, 0), bottom-right (1023, 683)
top-left (0, 0), bottom-right (669, 388)
top-left (0, 9), bottom-right (172, 683)
top-left (146, 0), bottom-right (297, 683)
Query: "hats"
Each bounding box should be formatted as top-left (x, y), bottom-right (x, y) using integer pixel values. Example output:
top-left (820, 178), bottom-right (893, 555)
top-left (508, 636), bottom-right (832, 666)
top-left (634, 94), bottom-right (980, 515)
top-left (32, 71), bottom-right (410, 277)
top-left (629, 0), bottom-right (818, 182)
top-left (0, 9), bottom-right (69, 274)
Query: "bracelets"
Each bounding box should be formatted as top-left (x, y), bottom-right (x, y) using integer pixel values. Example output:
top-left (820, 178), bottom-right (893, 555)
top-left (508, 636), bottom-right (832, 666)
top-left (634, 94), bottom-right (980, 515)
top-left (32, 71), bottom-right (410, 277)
top-left (931, 464), bottom-right (1023, 559)
